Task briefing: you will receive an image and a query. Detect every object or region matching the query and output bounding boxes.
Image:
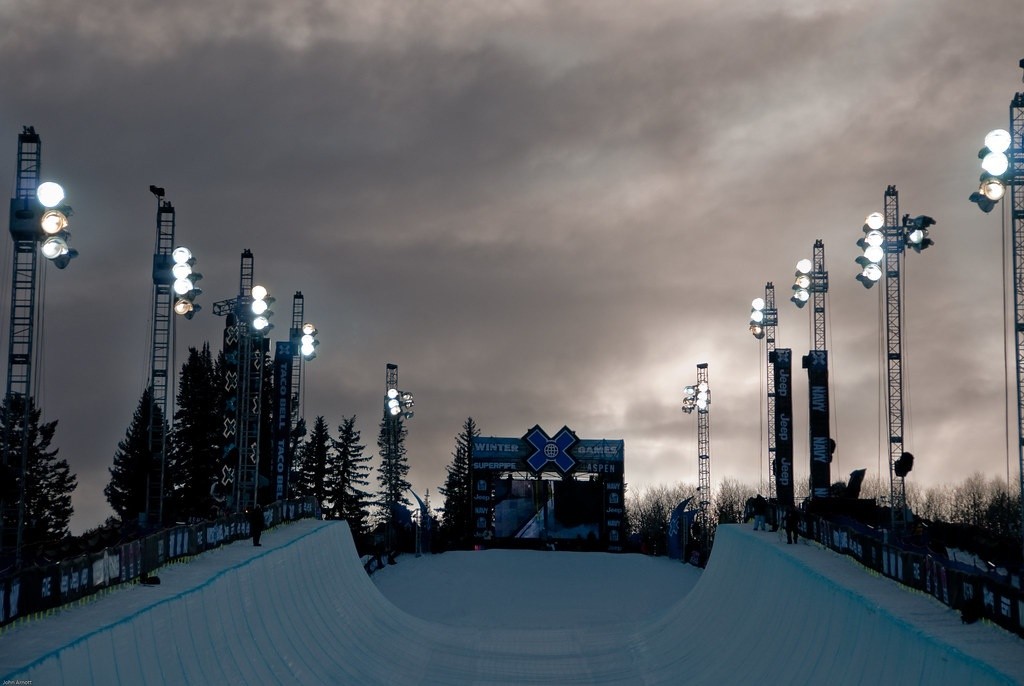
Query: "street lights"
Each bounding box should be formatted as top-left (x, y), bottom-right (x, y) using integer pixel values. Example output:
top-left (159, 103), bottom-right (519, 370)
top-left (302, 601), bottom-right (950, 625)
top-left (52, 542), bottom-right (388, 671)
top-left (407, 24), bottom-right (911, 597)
top-left (749, 282), bottom-right (794, 507)
top-left (968, 59), bottom-right (1024, 508)
top-left (213, 248), bottom-right (320, 515)
top-left (682, 363), bottom-right (712, 556)
top-left (791, 239), bottom-right (836, 508)
top-left (386, 364), bottom-right (415, 565)
top-left (0, 125), bottom-right (79, 573)
top-left (855, 185), bottom-right (937, 539)
top-left (139, 185), bottom-right (203, 536)
